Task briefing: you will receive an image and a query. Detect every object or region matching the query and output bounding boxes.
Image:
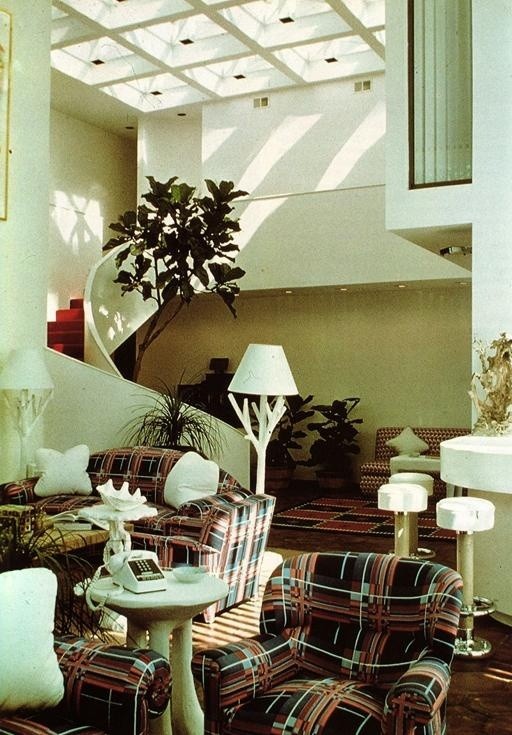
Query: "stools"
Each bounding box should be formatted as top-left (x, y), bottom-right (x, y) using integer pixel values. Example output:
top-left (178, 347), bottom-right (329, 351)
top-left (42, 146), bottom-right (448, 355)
top-left (377, 473), bottom-right (496, 658)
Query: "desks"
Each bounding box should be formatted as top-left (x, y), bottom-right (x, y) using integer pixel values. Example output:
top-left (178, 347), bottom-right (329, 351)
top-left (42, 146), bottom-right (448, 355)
top-left (390, 454), bottom-right (464, 498)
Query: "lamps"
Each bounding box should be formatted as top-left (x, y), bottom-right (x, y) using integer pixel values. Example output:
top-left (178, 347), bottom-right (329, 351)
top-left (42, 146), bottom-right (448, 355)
top-left (229, 343), bottom-right (299, 498)
top-left (0, 349), bottom-right (55, 480)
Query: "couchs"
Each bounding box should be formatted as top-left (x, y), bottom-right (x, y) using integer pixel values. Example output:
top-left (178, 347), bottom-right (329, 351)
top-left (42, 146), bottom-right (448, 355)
top-left (360, 428), bottom-right (470, 500)
top-left (2, 445), bottom-right (278, 625)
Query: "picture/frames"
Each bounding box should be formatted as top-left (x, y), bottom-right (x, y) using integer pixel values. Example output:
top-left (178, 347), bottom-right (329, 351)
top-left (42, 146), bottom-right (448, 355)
top-left (0, 9), bottom-right (13, 221)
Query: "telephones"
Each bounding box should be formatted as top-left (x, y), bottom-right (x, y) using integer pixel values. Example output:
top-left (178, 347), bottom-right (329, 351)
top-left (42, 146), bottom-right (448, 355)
top-left (104, 549), bottom-right (168, 595)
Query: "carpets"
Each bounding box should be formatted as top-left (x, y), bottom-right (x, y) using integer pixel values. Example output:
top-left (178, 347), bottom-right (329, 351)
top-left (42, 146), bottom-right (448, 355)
top-left (273, 498), bottom-right (456, 542)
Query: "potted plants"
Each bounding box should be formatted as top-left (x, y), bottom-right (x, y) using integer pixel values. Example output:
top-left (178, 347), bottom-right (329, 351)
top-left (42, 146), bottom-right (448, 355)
top-left (253, 395), bottom-right (364, 491)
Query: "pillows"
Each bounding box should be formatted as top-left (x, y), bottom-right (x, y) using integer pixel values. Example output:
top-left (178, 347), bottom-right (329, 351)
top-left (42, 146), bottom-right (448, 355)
top-left (32, 443), bottom-right (93, 498)
top-left (384, 429), bottom-right (428, 457)
top-left (162, 450), bottom-right (220, 510)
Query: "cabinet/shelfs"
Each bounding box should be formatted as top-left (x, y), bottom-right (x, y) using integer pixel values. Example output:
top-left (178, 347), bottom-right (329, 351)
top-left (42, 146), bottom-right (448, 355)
top-left (178, 373), bottom-right (245, 428)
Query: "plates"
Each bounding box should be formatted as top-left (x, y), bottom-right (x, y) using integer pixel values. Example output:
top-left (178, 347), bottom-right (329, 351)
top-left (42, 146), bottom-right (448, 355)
top-left (172, 565), bottom-right (209, 582)
